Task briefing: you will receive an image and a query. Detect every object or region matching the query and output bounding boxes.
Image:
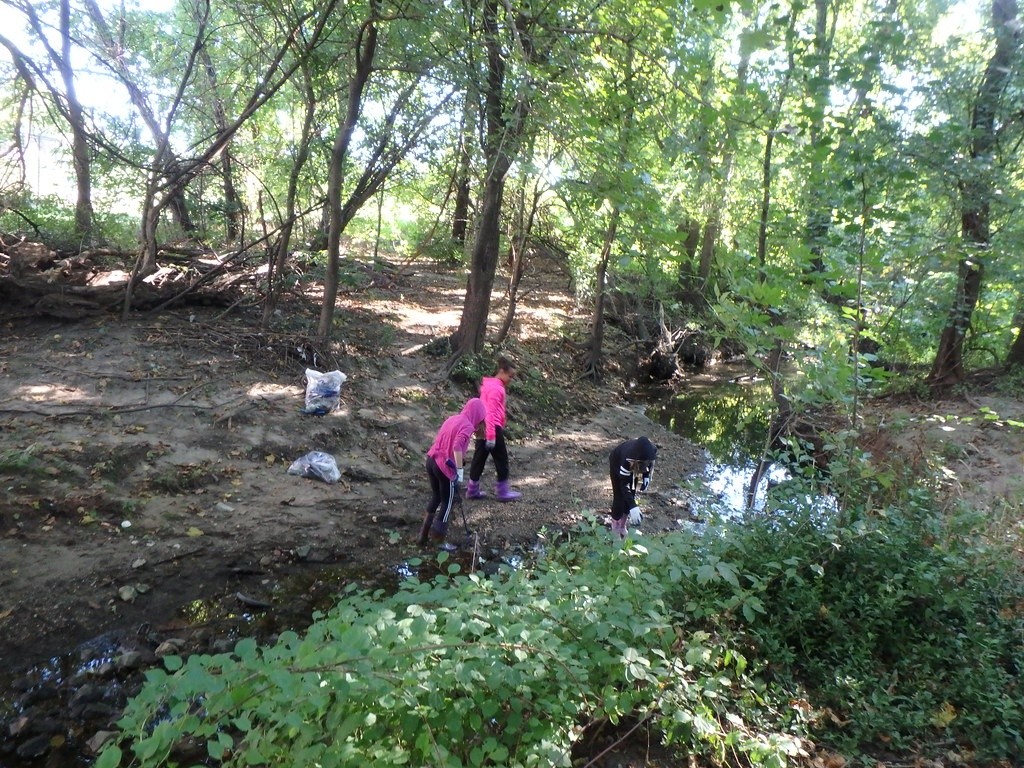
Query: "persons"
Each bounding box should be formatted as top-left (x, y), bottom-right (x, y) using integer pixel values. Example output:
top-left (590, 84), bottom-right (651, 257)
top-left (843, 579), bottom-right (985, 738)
top-left (609, 435), bottom-right (658, 540)
top-left (465, 356), bottom-right (524, 500)
top-left (415, 398), bottom-right (488, 551)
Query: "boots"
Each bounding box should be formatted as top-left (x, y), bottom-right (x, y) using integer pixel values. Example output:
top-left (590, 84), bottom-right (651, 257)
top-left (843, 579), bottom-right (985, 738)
top-left (620, 513), bottom-right (629, 536)
top-left (611, 518), bottom-right (622, 542)
top-left (465, 479), bottom-right (487, 499)
top-left (415, 510), bottom-right (435, 547)
top-left (431, 519), bottom-right (458, 551)
top-left (495, 480), bottom-right (521, 501)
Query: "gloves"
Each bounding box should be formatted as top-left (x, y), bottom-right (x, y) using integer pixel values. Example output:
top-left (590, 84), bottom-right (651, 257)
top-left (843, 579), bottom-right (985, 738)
top-left (503, 428), bottom-right (513, 441)
top-left (455, 468), bottom-right (465, 490)
top-left (629, 506), bottom-right (643, 526)
top-left (639, 478), bottom-right (649, 491)
top-left (486, 440), bottom-right (496, 448)
